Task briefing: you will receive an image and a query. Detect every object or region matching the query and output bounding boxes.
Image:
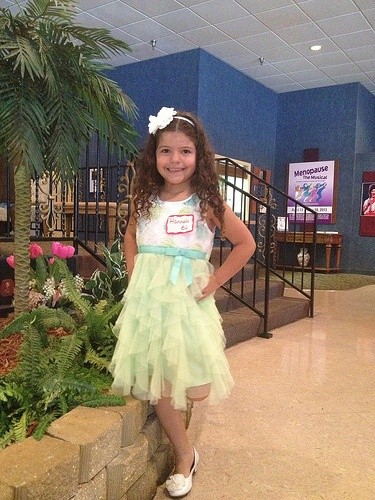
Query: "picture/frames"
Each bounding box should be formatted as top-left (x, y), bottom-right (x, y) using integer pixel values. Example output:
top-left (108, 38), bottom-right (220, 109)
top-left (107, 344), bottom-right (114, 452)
top-left (360, 181), bottom-right (375, 217)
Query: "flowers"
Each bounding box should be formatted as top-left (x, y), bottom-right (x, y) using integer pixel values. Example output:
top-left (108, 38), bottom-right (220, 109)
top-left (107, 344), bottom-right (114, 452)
top-left (147, 106), bottom-right (177, 135)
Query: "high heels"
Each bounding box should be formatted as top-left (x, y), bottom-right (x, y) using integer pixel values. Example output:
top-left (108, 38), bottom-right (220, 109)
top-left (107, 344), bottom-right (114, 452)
top-left (165, 443), bottom-right (199, 497)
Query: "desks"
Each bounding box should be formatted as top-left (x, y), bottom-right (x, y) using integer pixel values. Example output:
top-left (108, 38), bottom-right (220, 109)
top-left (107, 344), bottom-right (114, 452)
top-left (50, 201), bottom-right (130, 255)
top-left (271, 230), bottom-right (344, 275)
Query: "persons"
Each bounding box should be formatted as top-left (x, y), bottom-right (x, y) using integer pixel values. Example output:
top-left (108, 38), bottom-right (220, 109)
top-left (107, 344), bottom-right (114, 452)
top-left (107, 106), bottom-right (258, 498)
top-left (363, 184), bottom-right (375, 215)
